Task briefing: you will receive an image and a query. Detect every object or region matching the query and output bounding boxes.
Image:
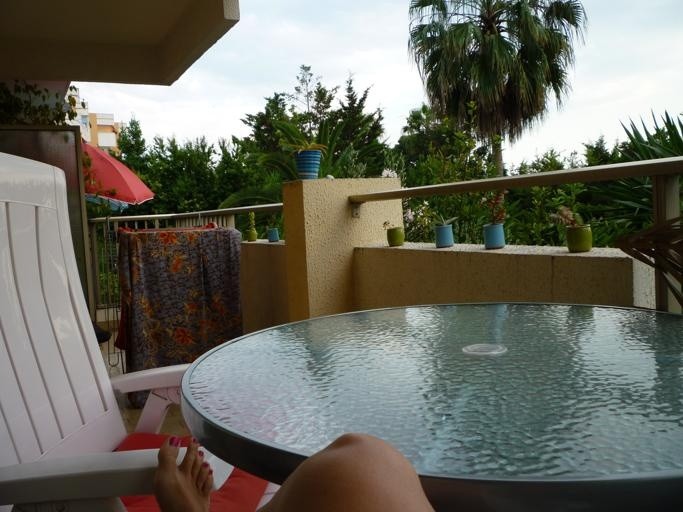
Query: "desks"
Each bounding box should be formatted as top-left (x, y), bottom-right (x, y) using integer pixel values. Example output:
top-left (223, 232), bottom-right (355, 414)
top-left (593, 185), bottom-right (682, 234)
top-left (178, 301), bottom-right (683, 511)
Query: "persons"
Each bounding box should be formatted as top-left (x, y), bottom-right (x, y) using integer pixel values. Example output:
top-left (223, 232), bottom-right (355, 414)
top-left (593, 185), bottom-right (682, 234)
top-left (151, 429), bottom-right (435, 512)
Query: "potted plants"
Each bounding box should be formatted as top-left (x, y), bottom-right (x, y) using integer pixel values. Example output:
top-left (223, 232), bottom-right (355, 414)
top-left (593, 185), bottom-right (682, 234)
top-left (285, 138), bottom-right (329, 179)
top-left (382, 219), bottom-right (406, 247)
top-left (431, 212), bottom-right (461, 248)
top-left (550, 205), bottom-right (594, 252)
top-left (478, 188), bottom-right (510, 250)
top-left (246, 211), bottom-right (257, 242)
top-left (267, 216), bottom-right (280, 242)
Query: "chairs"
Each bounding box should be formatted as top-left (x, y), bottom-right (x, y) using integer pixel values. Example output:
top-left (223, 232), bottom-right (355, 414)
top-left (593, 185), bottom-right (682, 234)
top-left (0, 152), bottom-right (281, 512)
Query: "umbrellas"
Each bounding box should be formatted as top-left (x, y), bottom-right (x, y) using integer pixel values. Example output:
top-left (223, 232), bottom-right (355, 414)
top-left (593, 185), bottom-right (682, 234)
top-left (80, 139), bottom-right (157, 206)
